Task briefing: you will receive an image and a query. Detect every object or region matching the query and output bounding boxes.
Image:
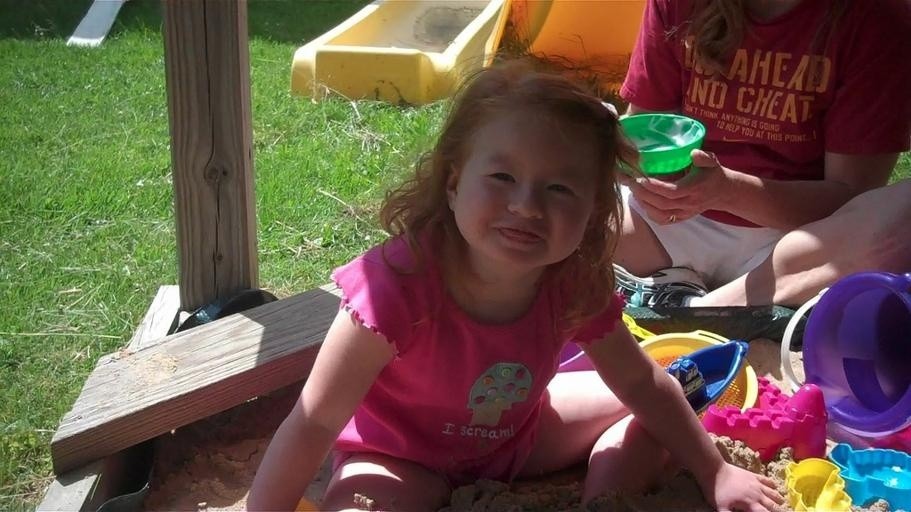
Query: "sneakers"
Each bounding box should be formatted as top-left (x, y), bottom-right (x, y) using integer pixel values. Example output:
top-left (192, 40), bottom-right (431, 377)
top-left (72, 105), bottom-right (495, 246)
top-left (608, 263), bottom-right (704, 316)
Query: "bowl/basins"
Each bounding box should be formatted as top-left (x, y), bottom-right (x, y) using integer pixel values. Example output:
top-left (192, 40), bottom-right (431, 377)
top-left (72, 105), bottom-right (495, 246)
top-left (615, 113), bottom-right (706, 176)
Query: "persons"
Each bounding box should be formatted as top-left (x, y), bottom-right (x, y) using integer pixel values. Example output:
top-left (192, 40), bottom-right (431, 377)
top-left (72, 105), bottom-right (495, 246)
top-left (600, 0), bottom-right (909, 311)
top-left (243, 48), bottom-right (783, 512)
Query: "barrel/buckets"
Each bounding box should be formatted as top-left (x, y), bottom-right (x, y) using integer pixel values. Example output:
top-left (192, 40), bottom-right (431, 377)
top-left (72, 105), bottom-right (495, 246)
top-left (779, 272), bottom-right (910, 434)
